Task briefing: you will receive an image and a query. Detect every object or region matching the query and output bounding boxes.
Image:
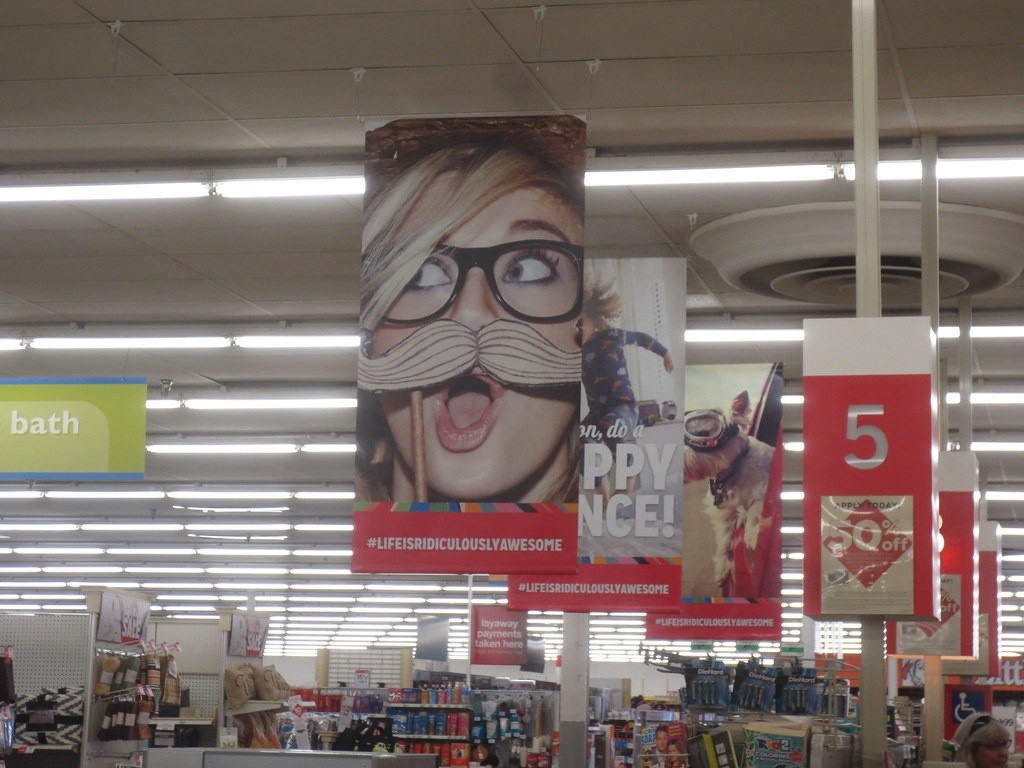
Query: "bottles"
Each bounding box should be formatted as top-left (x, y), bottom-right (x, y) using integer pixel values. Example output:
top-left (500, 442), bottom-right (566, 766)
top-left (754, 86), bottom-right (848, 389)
top-left (422, 681), bottom-right (470, 704)
top-left (389, 709), bottom-right (470, 735)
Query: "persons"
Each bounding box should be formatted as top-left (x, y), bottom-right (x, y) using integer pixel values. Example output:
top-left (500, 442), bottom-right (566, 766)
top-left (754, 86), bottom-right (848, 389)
top-left (952, 713), bottom-right (1014, 768)
top-left (644, 725), bottom-right (687, 768)
top-left (573, 275), bottom-right (672, 511)
top-left (361, 136), bottom-right (585, 512)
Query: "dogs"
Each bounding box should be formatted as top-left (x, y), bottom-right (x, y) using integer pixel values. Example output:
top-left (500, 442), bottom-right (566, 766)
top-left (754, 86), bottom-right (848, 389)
top-left (682, 390), bottom-right (776, 551)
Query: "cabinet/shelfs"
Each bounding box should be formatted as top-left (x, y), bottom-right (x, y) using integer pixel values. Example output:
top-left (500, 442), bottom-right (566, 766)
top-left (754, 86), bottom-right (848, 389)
top-left (151, 700), bottom-right (473, 742)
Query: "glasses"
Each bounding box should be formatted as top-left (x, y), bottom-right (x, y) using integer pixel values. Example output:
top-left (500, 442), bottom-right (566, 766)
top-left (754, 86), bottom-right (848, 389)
top-left (381, 241), bottom-right (583, 324)
top-left (976, 739), bottom-right (1012, 751)
top-left (969, 715), bottom-right (994, 736)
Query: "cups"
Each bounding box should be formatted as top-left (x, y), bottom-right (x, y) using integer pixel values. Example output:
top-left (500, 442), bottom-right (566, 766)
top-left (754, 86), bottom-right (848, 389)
top-left (403, 688), bottom-right (421, 703)
